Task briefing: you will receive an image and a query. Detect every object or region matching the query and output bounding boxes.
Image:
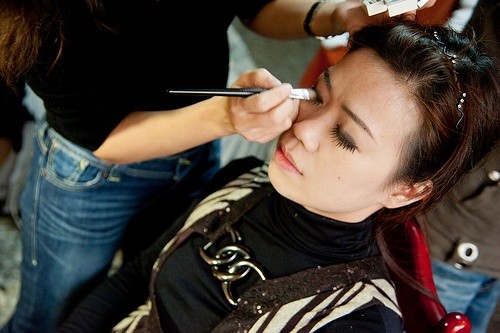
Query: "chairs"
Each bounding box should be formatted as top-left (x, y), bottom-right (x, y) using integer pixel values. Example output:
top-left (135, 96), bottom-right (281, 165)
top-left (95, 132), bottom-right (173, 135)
top-left (384, 212), bottom-right (472, 333)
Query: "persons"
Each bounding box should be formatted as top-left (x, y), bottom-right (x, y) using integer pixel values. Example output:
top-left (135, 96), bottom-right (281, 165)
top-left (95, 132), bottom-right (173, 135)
top-left (9, 0), bottom-right (368, 333)
top-left (57, 26), bottom-right (500, 333)
top-left (415, 9), bottom-right (500, 333)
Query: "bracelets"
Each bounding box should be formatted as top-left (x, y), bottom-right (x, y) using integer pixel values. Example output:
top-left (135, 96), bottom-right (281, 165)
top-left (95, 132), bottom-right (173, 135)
top-left (303, 2), bottom-right (328, 37)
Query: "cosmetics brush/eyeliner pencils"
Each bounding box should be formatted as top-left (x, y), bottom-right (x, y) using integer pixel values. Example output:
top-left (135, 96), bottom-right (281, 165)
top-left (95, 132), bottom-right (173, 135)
top-left (169, 86), bottom-right (315, 101)
